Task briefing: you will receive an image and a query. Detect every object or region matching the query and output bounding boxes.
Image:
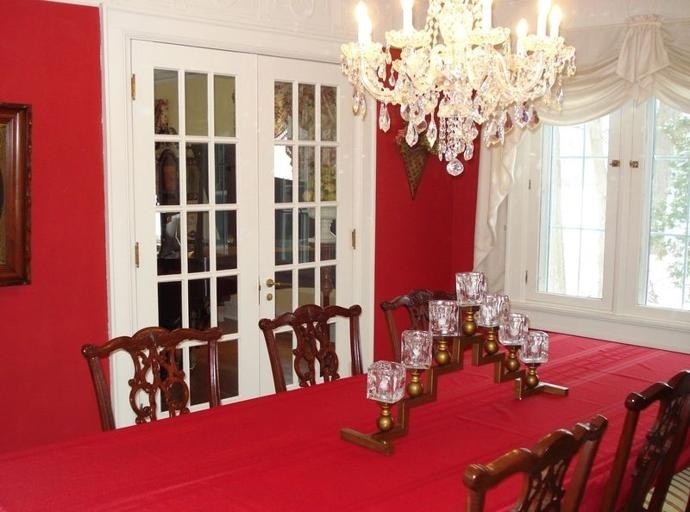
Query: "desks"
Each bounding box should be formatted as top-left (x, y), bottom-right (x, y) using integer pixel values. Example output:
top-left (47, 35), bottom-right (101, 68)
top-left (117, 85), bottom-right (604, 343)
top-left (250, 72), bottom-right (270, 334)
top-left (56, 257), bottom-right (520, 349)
top-left (0, 327), bottom-right (689, 512)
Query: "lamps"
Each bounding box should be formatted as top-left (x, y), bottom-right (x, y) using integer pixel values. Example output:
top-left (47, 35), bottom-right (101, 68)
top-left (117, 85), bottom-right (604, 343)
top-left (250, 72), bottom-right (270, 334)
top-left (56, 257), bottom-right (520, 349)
top-left (339, 0), bottom-right (578, 178)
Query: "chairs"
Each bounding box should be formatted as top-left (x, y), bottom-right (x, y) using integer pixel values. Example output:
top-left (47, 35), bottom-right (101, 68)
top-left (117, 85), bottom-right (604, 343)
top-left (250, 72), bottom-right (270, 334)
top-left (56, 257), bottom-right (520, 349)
top-left (258, 305), bottom-right (363, 393)
top-left (82, 326), bottom-right (228, 431)
top-left (381, 292), bottom-right (456, 363)
top-left (464, 369), bottom-right (690, 512)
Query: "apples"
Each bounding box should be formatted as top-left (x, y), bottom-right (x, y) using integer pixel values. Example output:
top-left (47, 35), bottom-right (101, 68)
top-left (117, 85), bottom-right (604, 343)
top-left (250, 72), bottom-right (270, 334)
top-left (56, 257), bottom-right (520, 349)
top-left (302, 163), bottom-right (336, 201)
